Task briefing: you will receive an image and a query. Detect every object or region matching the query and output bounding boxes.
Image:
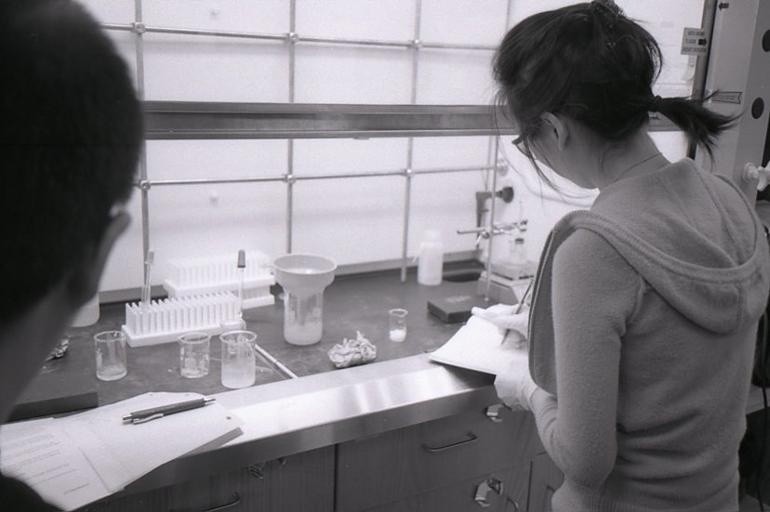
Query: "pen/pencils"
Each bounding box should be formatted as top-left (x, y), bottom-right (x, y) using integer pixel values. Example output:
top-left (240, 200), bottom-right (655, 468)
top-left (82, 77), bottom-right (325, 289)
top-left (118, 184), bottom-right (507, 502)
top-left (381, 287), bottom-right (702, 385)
top-left (255, 344), bottom-right (297, 378)
top-left (501, 279), bottom-right (533, 345)
top-left (122, 398), bottom-right (216, 424)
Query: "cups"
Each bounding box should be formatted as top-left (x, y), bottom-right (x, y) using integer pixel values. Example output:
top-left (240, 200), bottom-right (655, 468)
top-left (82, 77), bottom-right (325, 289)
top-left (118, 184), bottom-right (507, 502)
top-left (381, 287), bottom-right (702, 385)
top-left (415, 232), bottom-right (445, 286)
top-left (271, 255), bottom-right (337, 346)
top-left (69, 288), bottom-right (100, 328)
top-left (388, 308), bottom-right (409, 342)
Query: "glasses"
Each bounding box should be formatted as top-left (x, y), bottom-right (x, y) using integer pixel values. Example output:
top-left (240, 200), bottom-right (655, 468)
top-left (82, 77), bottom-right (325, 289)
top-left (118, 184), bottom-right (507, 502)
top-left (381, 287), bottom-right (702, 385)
top-left (512, 135), bottom-right (537, 160)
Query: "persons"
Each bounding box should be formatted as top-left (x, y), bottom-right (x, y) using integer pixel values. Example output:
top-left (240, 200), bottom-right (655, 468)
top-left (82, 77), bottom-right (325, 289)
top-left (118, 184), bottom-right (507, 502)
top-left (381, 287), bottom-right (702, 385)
top-left (0, 0), bottom-right (150, 510)
top-left (495, 3), bottom-right (767, 511)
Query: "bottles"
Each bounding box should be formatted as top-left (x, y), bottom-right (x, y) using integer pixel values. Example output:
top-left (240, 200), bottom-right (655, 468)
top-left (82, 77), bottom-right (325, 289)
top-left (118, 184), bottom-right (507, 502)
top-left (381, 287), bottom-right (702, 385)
top-left (93, 330), bottom-right (128, 382)
top-left (511, 238), bottom-right (524, 266)
top-left (176, 330), bottom-right (258, 389)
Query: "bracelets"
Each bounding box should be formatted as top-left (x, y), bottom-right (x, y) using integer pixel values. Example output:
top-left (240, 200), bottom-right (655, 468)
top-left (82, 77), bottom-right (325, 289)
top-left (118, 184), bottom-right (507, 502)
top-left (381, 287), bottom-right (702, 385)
top-left (526, 383), bottom-right (543, 417)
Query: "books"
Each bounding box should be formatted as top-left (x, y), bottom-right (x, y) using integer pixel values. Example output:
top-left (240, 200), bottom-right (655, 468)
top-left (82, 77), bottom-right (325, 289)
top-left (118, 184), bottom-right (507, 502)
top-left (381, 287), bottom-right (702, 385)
top-left (428, 300), bottom-right (530, 377)
top-left (1, 389), bottom-right (245, 511)
